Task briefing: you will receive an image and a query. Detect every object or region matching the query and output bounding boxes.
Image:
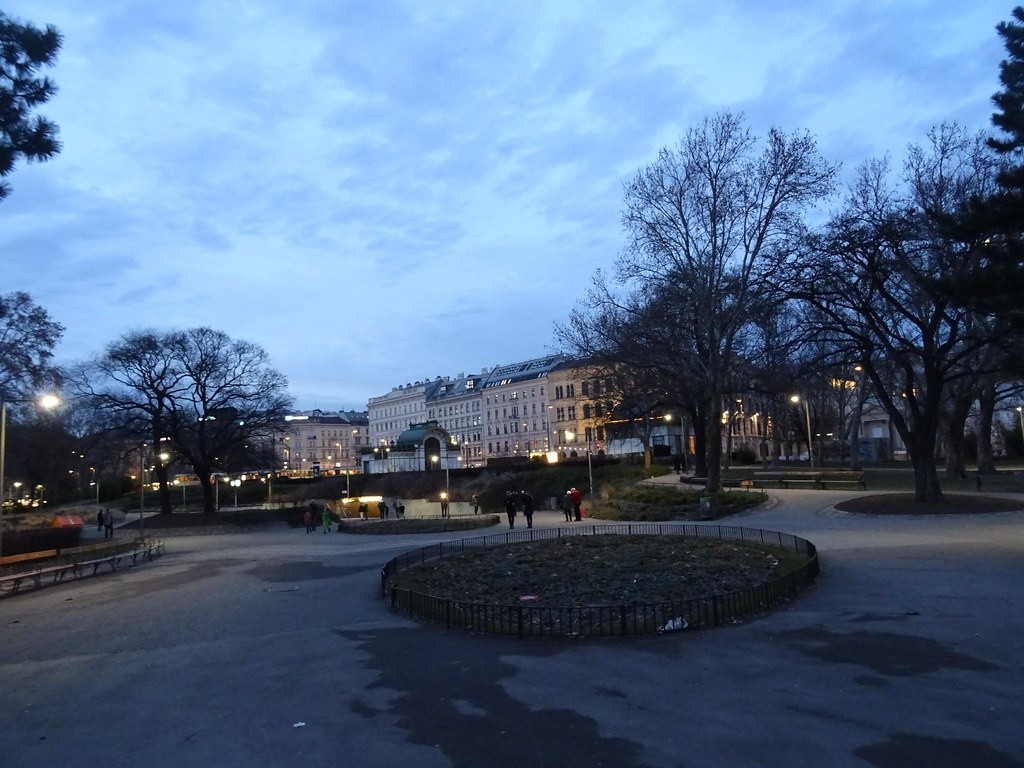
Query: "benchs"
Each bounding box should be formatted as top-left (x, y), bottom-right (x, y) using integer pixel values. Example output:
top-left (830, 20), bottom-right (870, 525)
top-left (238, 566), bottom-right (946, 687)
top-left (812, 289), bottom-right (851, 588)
top-left (752, 471), bottom-right (867, 491)
top-left (976, 474), bottom-right (1024, 490)
top-left (0, 533), bottom-right (166, 595)
top-left (680, 475), bottom-right (741, 487)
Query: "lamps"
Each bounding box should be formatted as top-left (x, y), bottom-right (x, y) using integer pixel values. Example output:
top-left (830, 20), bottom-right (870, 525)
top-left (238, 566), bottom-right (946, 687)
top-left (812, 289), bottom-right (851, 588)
top-left (390, 440), bottom-right (395, 445)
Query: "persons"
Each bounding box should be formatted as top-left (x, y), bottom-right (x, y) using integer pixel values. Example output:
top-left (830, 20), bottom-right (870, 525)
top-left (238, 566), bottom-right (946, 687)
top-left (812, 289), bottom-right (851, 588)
top-left (309, 503), bottom-right (318, 531)
top-left (364, 504), bottom-right (368, 520)
top-left (571, 487), bottom-right (582, 521)
top-left (440, 496), bottom-right (447, 517)
top-left (385, 506), bottom-right (389, 519)
top-left (562, 491), bottom-right (573, 521)
top-left (378, 499), bottom-right (386, 519)
top-left (104, 508), bottom-right (113, 539)
top-left (681, 452), bottom-right (686, 473)
top-left (393, 500), bottom-right (399, 518)
top-left (522, 491), bottom-right (533, 528)
top-left (505, 491), bottom-right (517, 529)
top-left (510, 491), bottom-right (519, 517)
top-left (359, 503), bottom-right (364, 521)
top-left (304, 510), bottom-right (312, 534)
top-left (472, 492), bottom-right (480, 515)
top-left (398, 502), bottom-right (406, 519)
top-left (321, 508), bottom-right (331, 534)
top-left (674, 454), bottom-right (681, 474)
top-left (97, 509), bottom-right (104, 531)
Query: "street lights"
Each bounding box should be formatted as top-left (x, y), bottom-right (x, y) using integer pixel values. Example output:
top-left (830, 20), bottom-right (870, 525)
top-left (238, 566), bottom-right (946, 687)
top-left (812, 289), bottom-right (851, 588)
top-left (0, 395), bottom-right (60, 515)
top-left (566, 432), bottom-right (593, 498)
top-left (432, 455), bottom-right (451, 519)
top-left (374, 448), bottom-right (396, 472)
top-left (792, 395), bottom-right (813, 470)
top-left (664, 413), bottom-right (690, 475)
top-left (90, 481), bottom-right (99, 503)
top-left (140, 453), bottom-right (170, 538)
top-left (214, 476), bottom-right (242, 510)
top-left (335, 443), bottom-right (341, 455)
